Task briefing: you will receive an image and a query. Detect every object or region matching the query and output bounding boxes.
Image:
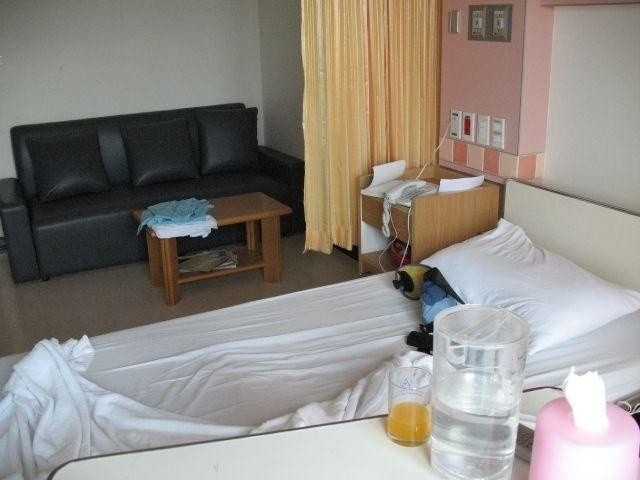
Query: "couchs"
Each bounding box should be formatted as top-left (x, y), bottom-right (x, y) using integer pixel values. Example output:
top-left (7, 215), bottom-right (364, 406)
top-left (1, 102), bottom-right (305, 287)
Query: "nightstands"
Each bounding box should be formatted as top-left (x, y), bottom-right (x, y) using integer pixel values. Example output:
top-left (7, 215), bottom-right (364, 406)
top-left (354, 164), bottom-right (499, 281)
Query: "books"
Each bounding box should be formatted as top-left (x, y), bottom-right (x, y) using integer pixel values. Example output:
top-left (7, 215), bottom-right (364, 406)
top-left (360, 160), bottom-right (407, 197)
top-left (177, 249), bottom-right (238, 273)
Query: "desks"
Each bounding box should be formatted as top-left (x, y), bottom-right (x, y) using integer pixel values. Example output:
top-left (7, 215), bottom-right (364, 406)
top-left (46, 381), bottom-right (571, 480)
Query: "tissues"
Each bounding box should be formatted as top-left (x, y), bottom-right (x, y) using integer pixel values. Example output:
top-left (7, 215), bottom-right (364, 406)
top-left (529, 369), bottom-right (640, 480)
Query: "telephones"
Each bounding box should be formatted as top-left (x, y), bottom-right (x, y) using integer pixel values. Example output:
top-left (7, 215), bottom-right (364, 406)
top-left (385, 179), bottom-right (439, 207)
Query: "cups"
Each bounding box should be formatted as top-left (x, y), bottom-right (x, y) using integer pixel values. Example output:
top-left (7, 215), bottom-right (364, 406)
top-left (387, 366), bottom-right (435, 446)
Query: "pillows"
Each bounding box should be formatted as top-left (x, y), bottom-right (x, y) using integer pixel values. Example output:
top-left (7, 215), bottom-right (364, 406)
top-left (419, 218), bottom-right (637, 363)
top-left (25, 106), bottom-right (266, 201)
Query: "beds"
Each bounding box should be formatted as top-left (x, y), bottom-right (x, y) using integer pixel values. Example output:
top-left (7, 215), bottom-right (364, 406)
top-left (1, 178), bottom-right (638, 480)
